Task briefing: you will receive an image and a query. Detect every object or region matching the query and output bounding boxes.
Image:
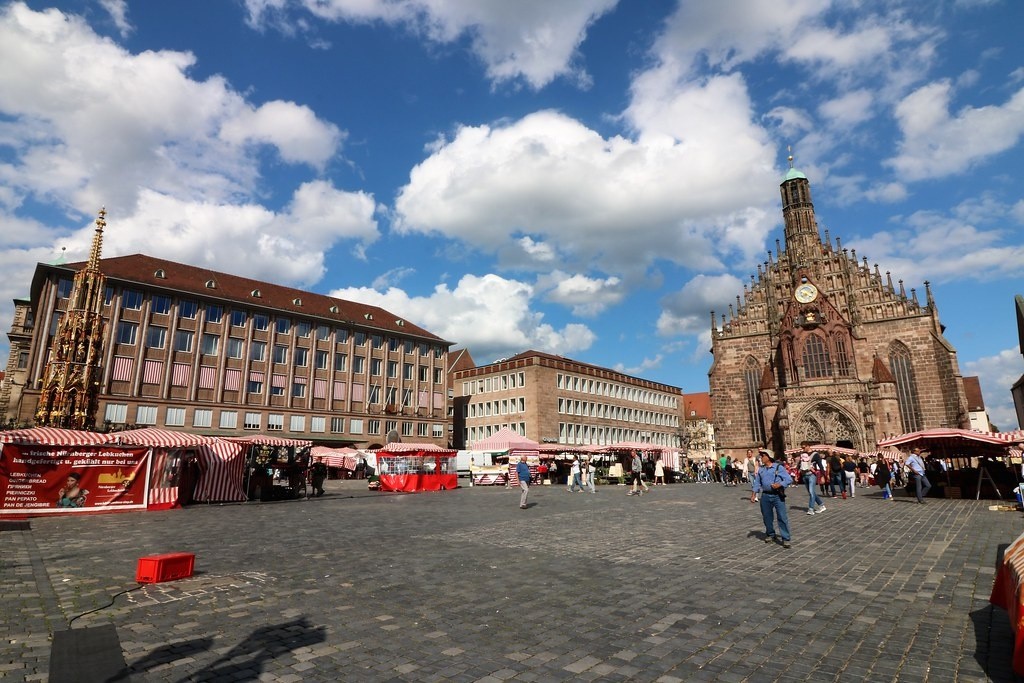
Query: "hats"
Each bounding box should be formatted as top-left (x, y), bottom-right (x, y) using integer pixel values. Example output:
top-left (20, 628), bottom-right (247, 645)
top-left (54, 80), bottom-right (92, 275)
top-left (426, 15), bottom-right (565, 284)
top-left (759, 449), bottom-right (775, 458)
top-left (801, 453), bottom-right (809, 462)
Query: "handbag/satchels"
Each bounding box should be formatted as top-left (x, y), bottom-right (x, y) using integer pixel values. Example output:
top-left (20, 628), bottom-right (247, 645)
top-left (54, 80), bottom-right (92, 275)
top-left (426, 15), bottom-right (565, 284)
top-left (776, 486), bottom-right (786, 502)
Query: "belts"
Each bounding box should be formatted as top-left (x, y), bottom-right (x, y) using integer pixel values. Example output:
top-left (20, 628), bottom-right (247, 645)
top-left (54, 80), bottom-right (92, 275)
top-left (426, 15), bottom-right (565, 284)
top-left (763, 490), bottom-right (778, 495)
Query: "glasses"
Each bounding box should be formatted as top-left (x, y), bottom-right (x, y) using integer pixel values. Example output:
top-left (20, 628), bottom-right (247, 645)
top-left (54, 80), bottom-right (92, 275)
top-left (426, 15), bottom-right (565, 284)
top-left (802, 446), bottom-right (807, 449)
top-left (761, 454), bottom-right (767, 458)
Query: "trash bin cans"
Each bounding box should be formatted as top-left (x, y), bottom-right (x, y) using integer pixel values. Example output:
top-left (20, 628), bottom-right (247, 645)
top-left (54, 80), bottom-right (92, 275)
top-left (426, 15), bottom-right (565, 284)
top-left (368, 476), bottom-right (379, 490)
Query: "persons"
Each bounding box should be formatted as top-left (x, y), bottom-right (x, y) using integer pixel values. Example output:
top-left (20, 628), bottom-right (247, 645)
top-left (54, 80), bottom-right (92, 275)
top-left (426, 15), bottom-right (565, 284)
top-left (538, 461), bottom-right (557, 484)
top-left (596, 457), bottom-right (603, 467)
top-left (651, 455), bottom-right (667, 486)
top-left (563, 452), bottom-right (595, 494)
top-left (56, 473), bottom-right (90, 508)
top-left (469, 458), bottom-right (475, 487)
top-left (624, 450), bottom-right (649, 496)
top-left (515, 456), bottom-right (531, 509)
top-left (363, 458), bottom-right (367, 479)
top-left (307, 456), bottom-right (325, 497)
top-left (679, 441), bottom-right (945, 550)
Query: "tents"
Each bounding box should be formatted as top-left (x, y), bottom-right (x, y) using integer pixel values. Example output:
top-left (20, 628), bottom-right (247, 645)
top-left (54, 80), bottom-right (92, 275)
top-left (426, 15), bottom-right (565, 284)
top-left (785, 427), bottom-right (1024, 459)
top-left (0, 426), bottom-right (458, 521)
top-left (539, 442), bottom-right (679, 471)
top-left (471, 426), bottom-right (539, 489)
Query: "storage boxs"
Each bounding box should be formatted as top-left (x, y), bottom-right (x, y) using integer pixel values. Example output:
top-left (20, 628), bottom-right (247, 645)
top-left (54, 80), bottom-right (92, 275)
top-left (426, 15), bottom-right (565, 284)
top-left (1015, 492), bottom-right (1022, 502)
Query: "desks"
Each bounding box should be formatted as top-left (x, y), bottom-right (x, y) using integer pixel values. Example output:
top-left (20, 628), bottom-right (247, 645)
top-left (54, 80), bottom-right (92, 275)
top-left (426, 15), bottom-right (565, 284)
top-left (599, 474), bottom-right (646, 486)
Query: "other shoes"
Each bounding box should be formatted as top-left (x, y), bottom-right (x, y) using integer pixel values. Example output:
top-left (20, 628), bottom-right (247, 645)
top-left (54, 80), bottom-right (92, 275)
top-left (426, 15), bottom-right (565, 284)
top-left (520, 505), bottom-right (528, 509)
top-left (782, 540), bottom-right (791, 548)
top-left (806, 508), bottom-right (814, 515)
top-left (830, 495), bottom-right (838, 499)
top-left (754, 498), bottom-right (759, 502)
top-left (765, 535), bottom-right (776, 542)
top-left (851, 493), bottom-right (854, 497)
top-left (917, 500), bottom-right (926, 504)
top-left (626, 488), bottom-right (649, 496)
top-left (663, 483), bottom-right (667, 485)
top-left (567, 488), bottom-right (596, 493)
top-left (888, 495), bottom-right (894, 500)
top-left (842, 491), bottom-right (846, 499)
top-left (815, 505), bottom-right (826, 513)
